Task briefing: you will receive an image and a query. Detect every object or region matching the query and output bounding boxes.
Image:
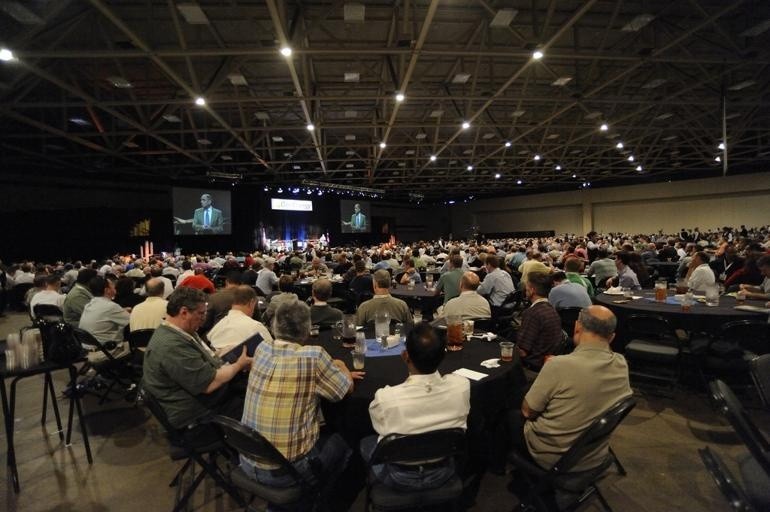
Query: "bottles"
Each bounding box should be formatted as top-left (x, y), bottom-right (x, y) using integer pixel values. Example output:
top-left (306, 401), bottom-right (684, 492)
top-left (395, 329), bottom-right (401, 338)
top-left (382, 336), bottom-right (388, 351)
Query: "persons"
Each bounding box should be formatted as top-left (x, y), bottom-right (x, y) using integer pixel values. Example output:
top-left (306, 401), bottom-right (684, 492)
top-left (192, 193), bottom-right (224, 234)
top-left (350, 202), bottom-right (367, 232)
top-left (1, 224), bottom-right (769, 491)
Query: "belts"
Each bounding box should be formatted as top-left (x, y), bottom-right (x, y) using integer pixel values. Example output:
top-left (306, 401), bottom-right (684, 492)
top-left (388, 456), bottom-right (450, 472)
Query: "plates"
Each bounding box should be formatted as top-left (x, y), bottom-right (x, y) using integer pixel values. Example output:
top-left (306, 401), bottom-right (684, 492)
top-left (603, 290), bottom-right (627, 295)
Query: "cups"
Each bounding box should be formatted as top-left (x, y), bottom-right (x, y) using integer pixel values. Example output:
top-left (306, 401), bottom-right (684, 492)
top-left (667, 258), bottom-right (672, 261)
top-left (336, 314), bottom-right (357, 347)
top-left (465, 320), bottom-right (475, 335)
top-left (350, 350), bottom-right (365, 370)
top-left (623, 288), bottom-right (631, 298)
top-left (446, 311), bottom-right (464, 351)
top-left (654, 280), bottom-right (668, 302)
top-left (499, 341), bottom-right (514, 363)
top-left (704, 282), bottom-right (720, 306)
top-left (375, 310), bottom-right (390, 344)
top-left (4, 326), bottom-right (43, 370)
top-left (425, 274), bottom-right (433, 288)
top-left (408, 279), bottom-right (415, 290)
top-left (355, 332), bottom-right (368, 353)
top-left (413, 314), bottom-right (423, 325)
